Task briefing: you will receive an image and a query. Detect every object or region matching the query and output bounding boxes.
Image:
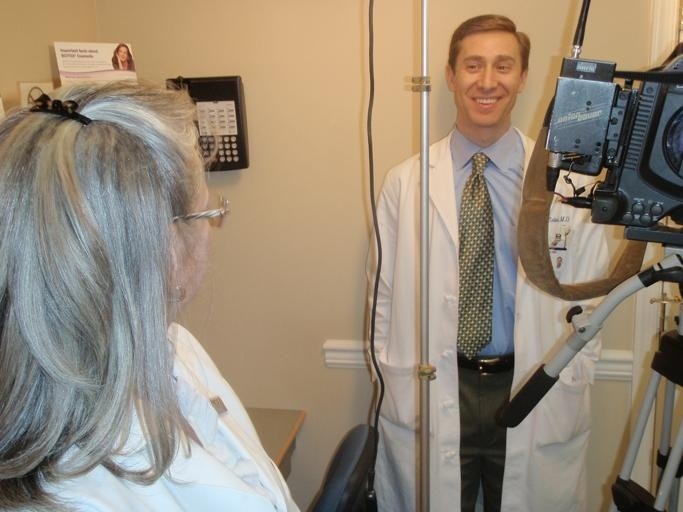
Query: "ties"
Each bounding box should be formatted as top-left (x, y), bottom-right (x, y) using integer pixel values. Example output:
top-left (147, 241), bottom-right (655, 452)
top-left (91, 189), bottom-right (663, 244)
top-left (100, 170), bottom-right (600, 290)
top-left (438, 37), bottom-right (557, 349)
top-left (457, 157), bottom-right (492, 359)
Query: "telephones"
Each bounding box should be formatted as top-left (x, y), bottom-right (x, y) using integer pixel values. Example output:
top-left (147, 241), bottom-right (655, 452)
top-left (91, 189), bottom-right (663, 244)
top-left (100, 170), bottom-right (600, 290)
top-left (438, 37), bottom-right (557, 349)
top-left (165, 76), bottom-right (249, 171)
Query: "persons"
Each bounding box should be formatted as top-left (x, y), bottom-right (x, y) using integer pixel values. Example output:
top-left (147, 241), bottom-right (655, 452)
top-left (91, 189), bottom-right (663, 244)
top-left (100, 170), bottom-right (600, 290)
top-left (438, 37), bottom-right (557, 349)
top-left (362, 14), bottom-right (609, 512)
top-left (2, 78), bottom-right (301, 512)
top-left (112, 44), bottom-right (134, 71)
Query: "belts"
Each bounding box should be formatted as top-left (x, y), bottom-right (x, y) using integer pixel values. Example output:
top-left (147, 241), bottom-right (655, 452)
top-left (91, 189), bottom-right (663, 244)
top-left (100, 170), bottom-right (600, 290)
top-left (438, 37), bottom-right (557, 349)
top-left (457, 356), bottom-right (511, 374)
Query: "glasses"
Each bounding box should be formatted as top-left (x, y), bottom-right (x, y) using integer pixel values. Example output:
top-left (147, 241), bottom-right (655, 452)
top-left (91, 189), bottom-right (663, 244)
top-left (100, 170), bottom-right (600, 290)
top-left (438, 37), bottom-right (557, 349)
top-left (167, 196), bottom-right (229, 229)
